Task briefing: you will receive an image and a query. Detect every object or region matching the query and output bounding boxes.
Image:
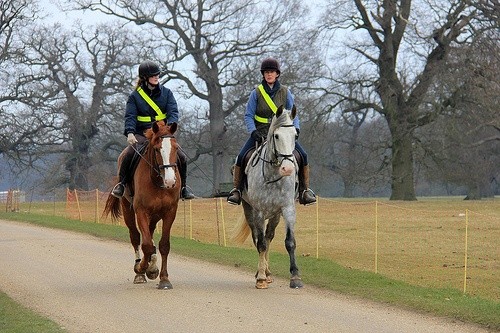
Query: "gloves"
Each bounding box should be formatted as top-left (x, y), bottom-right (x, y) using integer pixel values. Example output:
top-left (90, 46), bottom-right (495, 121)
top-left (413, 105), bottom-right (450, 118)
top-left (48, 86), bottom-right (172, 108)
top-left (127, 133), bottom-right (138, 145)
top-left (294, 128), bottom-right (300, 139)
top-left (251, 130), bottom-right (266, 142)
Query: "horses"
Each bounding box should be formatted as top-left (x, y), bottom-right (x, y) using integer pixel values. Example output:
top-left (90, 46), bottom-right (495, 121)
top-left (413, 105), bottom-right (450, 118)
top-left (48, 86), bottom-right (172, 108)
top-left (103, 121), bottom-right (182, 290)
top-left (231, 103), bottom-right (305, 289)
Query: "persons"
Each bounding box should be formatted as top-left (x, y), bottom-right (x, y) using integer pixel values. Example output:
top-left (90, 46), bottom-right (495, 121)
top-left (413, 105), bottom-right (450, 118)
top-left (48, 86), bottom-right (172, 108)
top-left (228, 58), bottom-right (316, 203)
top-left (113, 61), bottom-right (195, 199)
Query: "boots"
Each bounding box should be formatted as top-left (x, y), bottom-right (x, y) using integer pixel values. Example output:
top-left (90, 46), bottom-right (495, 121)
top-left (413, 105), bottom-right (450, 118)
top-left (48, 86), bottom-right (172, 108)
top-left (115, 155), bottom-right (133, 196)
top-left (297, 163), bottom-right (316, 204)
top-left (228, 164), bottom-right (244, 205)
top-left (177, 160), bottom-right (195, 199)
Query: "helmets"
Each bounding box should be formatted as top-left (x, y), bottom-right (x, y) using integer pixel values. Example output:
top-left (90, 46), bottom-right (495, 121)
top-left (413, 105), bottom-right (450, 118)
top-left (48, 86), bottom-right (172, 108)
top-left (138, 60), bottom-right (161, 78)
top-left (260, 59), bottom-right (281, 77)
